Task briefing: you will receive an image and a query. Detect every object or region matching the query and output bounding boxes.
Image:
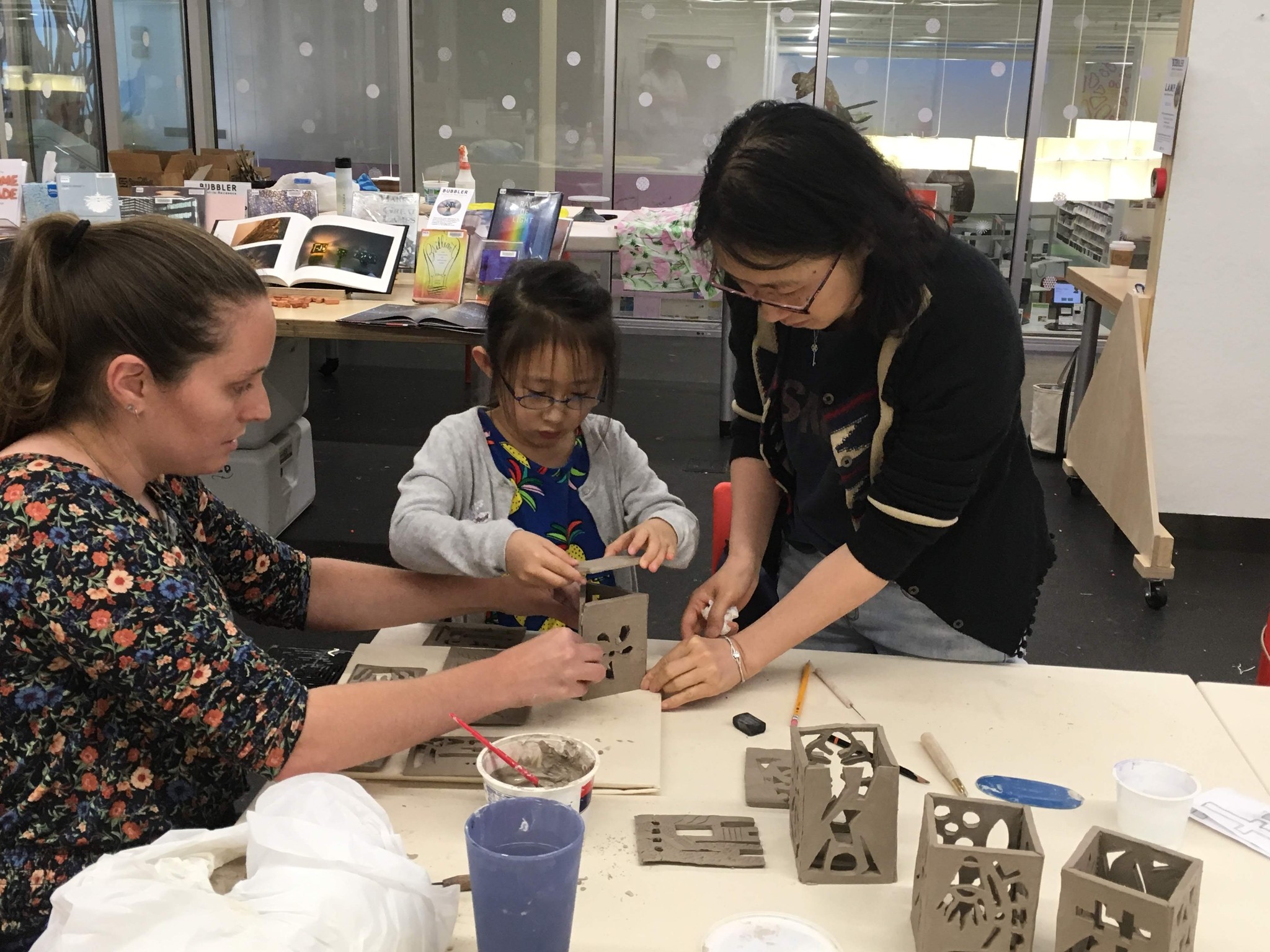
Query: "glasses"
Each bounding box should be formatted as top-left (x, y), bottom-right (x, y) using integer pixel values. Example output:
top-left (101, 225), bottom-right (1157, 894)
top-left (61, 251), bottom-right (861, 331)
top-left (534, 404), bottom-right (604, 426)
top-left (710, 246), bottom-right (848, 315)
top-left (492, 368), bottom-right (605, 411)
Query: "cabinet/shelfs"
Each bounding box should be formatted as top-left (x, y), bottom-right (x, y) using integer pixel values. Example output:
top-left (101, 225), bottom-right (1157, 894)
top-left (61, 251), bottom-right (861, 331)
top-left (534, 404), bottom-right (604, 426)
top-left (1057, 200), bottom-right (1126, 268)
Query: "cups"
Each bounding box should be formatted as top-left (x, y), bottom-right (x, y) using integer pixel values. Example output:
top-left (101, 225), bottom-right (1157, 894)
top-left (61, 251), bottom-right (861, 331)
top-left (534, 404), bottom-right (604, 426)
top-left (462, 798), bottom-right (585, 952)
top-left (1109, 240), bottom-right (1136, 280)
top-left (475, 732), bottom-right (601, 823)
top-left (422, 180), bottom-right (449, 206)
top-left (1111, 760), bottom-right (1200, 868)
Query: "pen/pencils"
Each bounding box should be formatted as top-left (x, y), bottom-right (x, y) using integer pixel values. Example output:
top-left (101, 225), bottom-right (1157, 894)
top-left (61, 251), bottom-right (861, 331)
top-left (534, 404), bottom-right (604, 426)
top-left (920, 732), bottom-right (968, 798)
top-left (826, 735), bottom-right (930, 785)
top-left (791, 659), bottom-right (811, 727)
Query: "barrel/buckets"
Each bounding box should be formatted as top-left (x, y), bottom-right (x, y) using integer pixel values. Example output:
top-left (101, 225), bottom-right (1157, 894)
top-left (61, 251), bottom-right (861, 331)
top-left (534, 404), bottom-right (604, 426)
top-left (1255, 608), bottom-right (1270, 687)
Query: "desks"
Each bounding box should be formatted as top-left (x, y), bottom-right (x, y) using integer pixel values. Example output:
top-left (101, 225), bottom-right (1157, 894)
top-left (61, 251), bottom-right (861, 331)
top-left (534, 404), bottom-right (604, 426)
top-left (556, 206), bottom-right (737, 440)
top-left (1063, 266), bottom-right (1146, 544)
top-left (270, 285), bottom-right (494, 408)
top-left (215, 621), bottom-right (1269, 952)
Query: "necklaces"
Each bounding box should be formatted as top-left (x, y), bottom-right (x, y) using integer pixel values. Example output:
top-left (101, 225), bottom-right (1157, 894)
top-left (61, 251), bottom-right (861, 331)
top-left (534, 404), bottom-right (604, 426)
top-left (808, 318), bottom-right (821, 367)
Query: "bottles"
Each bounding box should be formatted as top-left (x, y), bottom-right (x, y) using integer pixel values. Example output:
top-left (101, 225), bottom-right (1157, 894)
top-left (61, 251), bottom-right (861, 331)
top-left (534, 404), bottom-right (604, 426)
top-left (335, 156), bottom-right (353, 217)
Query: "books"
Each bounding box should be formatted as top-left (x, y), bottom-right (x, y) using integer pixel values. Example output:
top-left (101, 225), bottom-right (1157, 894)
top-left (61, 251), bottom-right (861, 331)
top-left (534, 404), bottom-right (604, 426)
top-left (209, 212), bottom-right (409, 295)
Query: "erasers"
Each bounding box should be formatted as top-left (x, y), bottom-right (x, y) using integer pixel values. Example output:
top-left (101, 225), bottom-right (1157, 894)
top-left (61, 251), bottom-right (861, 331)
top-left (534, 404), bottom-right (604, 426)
top-left (733, 712), bottom-right (766, 736)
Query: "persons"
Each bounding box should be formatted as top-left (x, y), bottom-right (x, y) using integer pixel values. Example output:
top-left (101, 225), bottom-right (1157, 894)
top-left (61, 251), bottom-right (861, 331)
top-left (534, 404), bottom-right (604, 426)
top-left (388, 260), bottom-right (700, 632)
top-left (0, 216), bottom-right (607, 951)
top-left (638, 101), bottom-right (1057, 713)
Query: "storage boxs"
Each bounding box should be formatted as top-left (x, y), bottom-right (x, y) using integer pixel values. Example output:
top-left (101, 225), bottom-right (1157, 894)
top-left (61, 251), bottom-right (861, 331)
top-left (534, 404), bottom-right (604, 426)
top-left (108, 146), bottom-right (250, 196)
top-left (198, 335), bottom-right (313, 541)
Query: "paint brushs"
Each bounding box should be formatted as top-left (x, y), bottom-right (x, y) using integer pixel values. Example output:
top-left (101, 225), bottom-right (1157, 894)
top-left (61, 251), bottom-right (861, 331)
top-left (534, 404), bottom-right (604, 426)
top-left (235, 144), bottom-right (266, 181)
top-left (449, 712), bottom-right (548, 788)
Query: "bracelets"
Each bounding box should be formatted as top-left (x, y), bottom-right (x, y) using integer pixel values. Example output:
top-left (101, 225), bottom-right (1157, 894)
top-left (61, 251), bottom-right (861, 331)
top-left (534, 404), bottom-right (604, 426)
top-left (719, 635), bottom-right (746, 683)
